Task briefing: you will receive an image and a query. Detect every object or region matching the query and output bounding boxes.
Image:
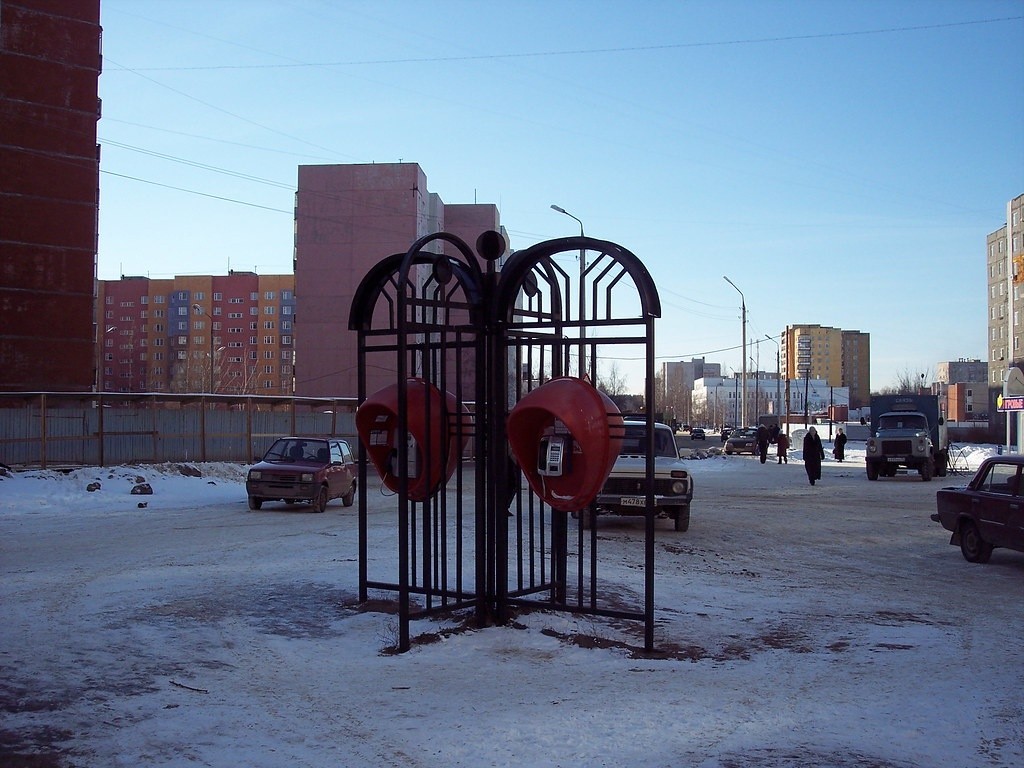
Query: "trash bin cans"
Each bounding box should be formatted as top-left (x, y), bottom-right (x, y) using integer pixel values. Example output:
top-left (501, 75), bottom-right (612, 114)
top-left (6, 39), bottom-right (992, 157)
top-left (995, 444), bottom-right (1003, 455)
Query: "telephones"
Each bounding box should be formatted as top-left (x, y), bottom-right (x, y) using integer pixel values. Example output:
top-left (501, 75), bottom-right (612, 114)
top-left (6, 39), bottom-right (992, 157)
top-left (536, 435), bottom-right (570, 477)
top-left (381, 427), bottom-right (421, 480)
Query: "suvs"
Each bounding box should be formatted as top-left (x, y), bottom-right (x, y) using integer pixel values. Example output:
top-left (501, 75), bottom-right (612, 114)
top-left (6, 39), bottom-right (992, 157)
top-left (570, 416), bottom-right (694, 533)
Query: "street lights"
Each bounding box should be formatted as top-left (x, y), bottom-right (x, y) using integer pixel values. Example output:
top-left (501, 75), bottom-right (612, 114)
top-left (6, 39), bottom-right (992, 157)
top-left (722, 275), bottom-right (747, 426)
top-left (550, 204), bottom-right (585, 379)
top-left (765, 334), bottom-right (781, 430)
top-left (192, 303), bottom-right (214, 395)
top-left (750, 356), bottom-right (759, 426)
top-left (730, 367), bottom-right (737, 427)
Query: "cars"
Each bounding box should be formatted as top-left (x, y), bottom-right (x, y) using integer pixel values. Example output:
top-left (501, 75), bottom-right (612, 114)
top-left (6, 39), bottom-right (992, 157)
top-left (675, 423), bottom-right (690, 432)
top-left (691, 427), bottom-right (706, 441)
top-left (724, 426), bottom-right (761, 456)
top-left (246, 436), bottom-right (359, 513)
top-left (720, 424), bottom-right (736, 442)
top-left (930, 455), bottom-right (1024, 564)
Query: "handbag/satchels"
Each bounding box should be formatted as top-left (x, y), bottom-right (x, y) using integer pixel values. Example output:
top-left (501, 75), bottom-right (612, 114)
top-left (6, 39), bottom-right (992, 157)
top-left (833, 449), bottom-right (836, 455)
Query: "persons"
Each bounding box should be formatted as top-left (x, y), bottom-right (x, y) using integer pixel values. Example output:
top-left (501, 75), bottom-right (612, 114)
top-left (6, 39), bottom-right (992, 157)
top-left (803, 427), bottom-right (825, 486)
top-left (768, 423), bottom-right (780, 441)
top-left (834, 428), bottom-right (847, 462)
top-left (689, 425), bottom-right (692, 435)
top-left (755, 423), bottom-right (771, 464)
top-left (771, 429), bottom-right (790, 465)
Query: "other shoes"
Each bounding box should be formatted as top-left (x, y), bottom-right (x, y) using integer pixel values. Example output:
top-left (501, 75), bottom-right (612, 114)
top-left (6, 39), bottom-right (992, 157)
top-left (811, 480), bottom-right (815, 486)
top-left (837, 460), bottom-right (842, 462)
top-left (507, 509), bottom-right (514, 517)
top-left (778, 462), bottom-right (782, 464)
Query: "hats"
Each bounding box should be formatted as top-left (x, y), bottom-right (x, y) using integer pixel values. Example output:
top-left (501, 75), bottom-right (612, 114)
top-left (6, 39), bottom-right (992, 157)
top-left (779, 429), bottom-right (784, 435)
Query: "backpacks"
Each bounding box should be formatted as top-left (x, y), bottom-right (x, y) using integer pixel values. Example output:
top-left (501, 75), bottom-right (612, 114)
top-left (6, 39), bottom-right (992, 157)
top-left (759, 428), bottom-right (767, 440)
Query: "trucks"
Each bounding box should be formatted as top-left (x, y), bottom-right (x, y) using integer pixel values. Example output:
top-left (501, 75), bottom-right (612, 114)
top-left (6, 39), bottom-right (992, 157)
top-left (860, 393), bottom-right (951, 482)
